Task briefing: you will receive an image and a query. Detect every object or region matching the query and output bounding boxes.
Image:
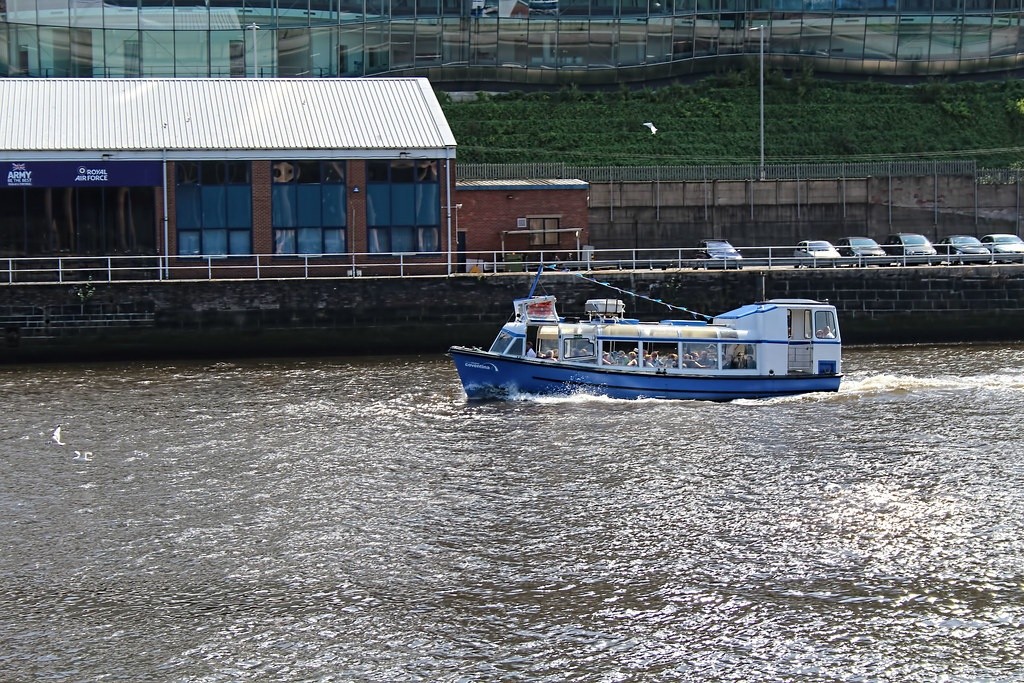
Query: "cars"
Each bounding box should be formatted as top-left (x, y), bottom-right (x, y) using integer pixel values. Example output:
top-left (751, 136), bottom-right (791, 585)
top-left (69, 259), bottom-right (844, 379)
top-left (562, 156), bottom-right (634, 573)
top-left (883, 232), bottom-right (937, 266)
top-left (979, 234), bottom-right (1024, 264)
top-left (835, 237), bottom-right (888, 268)
top-left (937, 235), bottom-right (990, 266)
top-left (793, 240), bottom-right (843, 269)
top-left (692, 238), bottom-right (743, 270)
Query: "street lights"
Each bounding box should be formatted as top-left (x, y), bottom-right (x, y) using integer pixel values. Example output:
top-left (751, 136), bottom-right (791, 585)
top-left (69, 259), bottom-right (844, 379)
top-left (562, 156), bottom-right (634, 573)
top-left (748, 24), bottom-right (765, 181)
top-left (246, 21), bottom-right (259, 78)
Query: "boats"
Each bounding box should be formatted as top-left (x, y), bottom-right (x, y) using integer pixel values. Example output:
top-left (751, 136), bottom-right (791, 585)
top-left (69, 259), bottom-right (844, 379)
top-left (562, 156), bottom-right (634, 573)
top-left (449, 261), bottom-right (843, 404)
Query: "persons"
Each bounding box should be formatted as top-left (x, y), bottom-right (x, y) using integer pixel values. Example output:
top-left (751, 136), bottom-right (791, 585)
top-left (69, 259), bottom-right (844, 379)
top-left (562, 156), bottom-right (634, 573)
top-left (525, 342), bottom-right (536, 358)
top-left (601, 348), bottom-right (664, 369)
top-left (543, 347), bottom-right (593, 363)
top-left (666, 351), bottom-right (754, 370)
